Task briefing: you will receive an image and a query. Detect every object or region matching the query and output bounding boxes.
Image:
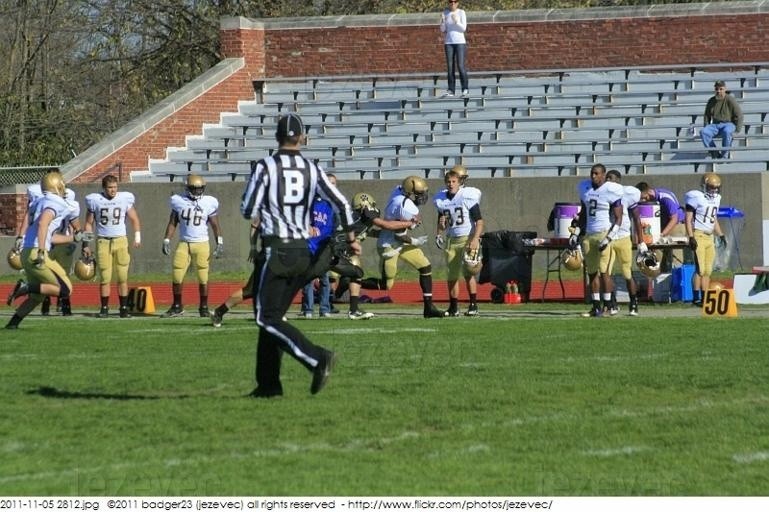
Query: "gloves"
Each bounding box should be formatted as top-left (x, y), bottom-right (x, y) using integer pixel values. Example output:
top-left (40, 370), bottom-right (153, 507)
top-left (689, 236), bottom-right (698, 250)
top-left (412, 234), bottom-right (428, 248)
top-left (599, 236), bottom-right (611, 253)
top-left (73, 231), bottom-right (95, 242)
top-left (435, 235), bottom-right (444, 249)
top-left (719, 235), bottom-right (727, 250)
top-left (637, 242), bottom-right (648, 254)
top-left (30, 250), bottom-right (46, 266)
top-left (568, 233), bottom-right (578, 249)
top-left (162, 240), bottom-right (171, 255)
top-left (213, 244), bottom-right (225, 259)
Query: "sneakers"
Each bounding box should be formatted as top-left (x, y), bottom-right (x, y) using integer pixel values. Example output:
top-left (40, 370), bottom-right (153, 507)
top-left (581, 294), bottom-right (639, 319)
top-left (7, 279), bottom-right (29, 306)
top-left (244, 385), bottom-right (284, 398)
top-left (98, 305), bottom-right (132, 319)
top-left (423, 308), bottom-right (446, 319)
top-left (443, 305), bottom-right (459, 319)
top-left (464, 306), bottom-right (477, 316)
top-left (294, 302), bottom-right (374, 323)
top-left (311, 347), bottom-right (335, 395)
top-left (160, 305), bottom-right (223, 327)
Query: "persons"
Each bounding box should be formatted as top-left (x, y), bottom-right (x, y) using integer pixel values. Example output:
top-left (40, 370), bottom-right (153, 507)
top-left (2, 162), bottom-right (729, 329)
top-left (438, 0), bottom-right (471, 97)
top-left (700, 80), bottom-right (744, 159)
top-left (240, 114), bottom-right (364, 397)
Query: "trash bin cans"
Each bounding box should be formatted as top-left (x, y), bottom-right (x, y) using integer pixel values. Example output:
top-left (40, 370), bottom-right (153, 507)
top-left (481, 231), bottom-right (538, 303)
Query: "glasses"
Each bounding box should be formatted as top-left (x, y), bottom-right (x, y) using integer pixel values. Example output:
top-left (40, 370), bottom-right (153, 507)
top-left (715, 88), bottom-right (726, 90)
top-left (448, 1), bottom-right (458, 3)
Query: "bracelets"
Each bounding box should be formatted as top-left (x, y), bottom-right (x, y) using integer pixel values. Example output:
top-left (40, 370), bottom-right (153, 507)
top-left (346, 239), bottom-right (357, 246)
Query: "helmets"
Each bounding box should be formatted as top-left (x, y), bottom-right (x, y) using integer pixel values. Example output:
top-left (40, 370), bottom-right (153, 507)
top-left (352, 193), bottom-right (378, 215)
top-left (7, 249), bottom-right (24, 269)
top-left (41, 174), bottom-right (68, 197)
top-left (402, 176), bottom-right (428, 203)
top-left (636, 253), bottom-right (661, 276)
top-left (463, 251), bottom-right (483, 274)
top-left (562, 249), bottom-right (581, 270)
top-left (701, 173), bottom-right (721, 200)
top-left (451, 165), bottom-right (467, 182)
top-left (186, 174), bottom-right (207, 199)
top-left (75, 258), bottom-right (97, 280)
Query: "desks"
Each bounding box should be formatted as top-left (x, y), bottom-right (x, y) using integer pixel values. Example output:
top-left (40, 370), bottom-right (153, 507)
top-left (521, 240), bottom-right (693, 305)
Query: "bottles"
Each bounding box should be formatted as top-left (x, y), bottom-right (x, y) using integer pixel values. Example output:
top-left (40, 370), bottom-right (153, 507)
top-left (505, 281), bottom-right (519, 295)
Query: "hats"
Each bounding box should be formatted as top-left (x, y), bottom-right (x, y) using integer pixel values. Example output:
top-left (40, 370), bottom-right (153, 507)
top-left (275, 115), bottom-right (305, 138)
top-left (714, 80), bottom-right (726, 87)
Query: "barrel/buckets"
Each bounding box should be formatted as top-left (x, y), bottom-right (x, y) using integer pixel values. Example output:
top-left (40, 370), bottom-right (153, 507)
top-left (631, 200), bottom-right (661, 243)
top-left (554, 202), bottom-right (583, 238)
